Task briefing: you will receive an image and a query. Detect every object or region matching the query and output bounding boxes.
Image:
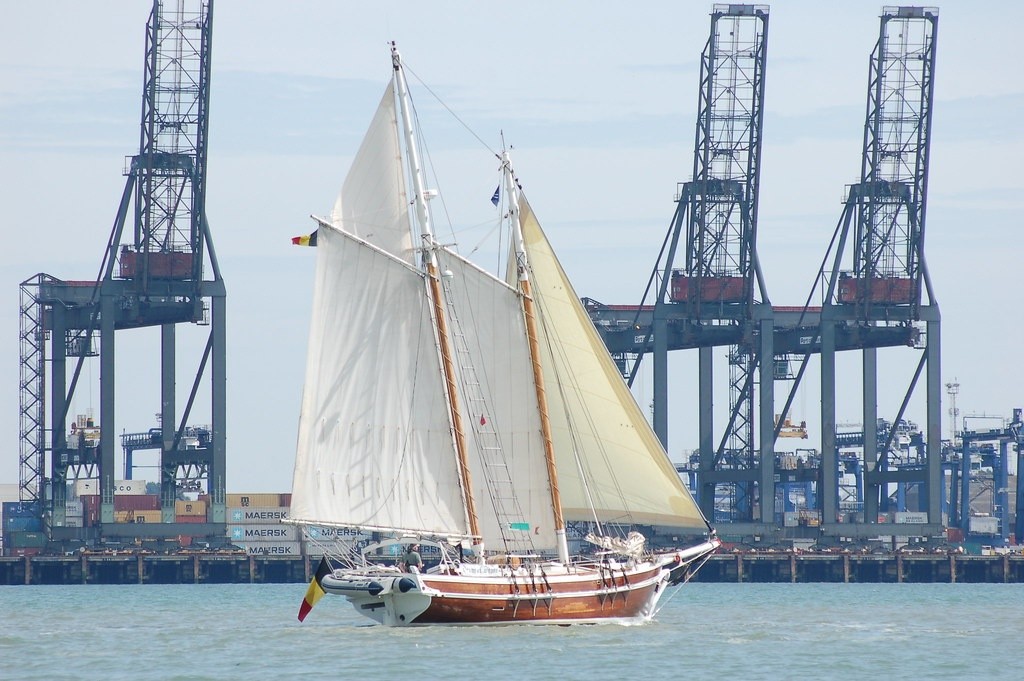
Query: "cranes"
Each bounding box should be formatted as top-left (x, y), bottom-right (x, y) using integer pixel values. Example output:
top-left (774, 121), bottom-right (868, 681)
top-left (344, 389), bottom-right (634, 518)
top-left (833, 3), bottom-right (940, 304)
top-left (670, 1), bottom-right (770, 302)
top-left (115, 0), bottom-right (214, 281)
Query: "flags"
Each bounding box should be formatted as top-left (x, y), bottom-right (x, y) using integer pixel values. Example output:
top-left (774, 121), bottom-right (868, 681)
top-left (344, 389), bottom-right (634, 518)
top-left (292, 229), bottom-right (317, 246)
top-left (491, 186), bottom-right (499, 206)
top-left (297, 557), bottom-right (333, 623)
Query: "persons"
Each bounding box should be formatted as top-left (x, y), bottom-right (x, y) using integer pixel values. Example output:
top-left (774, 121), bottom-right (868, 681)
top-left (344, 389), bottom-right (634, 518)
top-left (403, 543), bottom-right (422, 573)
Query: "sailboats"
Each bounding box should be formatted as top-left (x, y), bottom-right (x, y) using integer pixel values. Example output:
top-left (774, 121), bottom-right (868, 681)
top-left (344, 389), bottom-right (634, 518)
top-left (279, 41), bottom-right (722, 628)
